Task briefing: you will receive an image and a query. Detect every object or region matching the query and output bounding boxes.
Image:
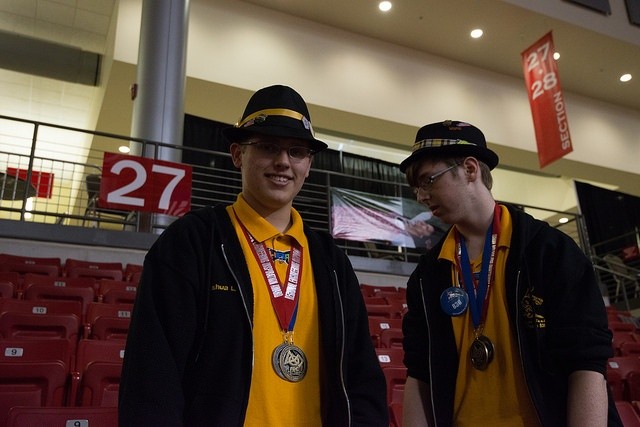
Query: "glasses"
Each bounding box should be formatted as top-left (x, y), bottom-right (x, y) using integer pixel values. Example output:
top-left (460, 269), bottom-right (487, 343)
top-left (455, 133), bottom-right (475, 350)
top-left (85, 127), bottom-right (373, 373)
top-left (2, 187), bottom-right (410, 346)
top-left (240, 140), bottom-right (315, 160)
top-left (409, 161), bottom-right (463, 197)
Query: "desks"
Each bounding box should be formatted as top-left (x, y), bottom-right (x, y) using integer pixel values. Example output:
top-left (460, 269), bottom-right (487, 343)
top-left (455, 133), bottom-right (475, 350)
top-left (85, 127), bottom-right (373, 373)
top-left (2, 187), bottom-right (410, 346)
top-left (0, 173), bottom-right (36, 201)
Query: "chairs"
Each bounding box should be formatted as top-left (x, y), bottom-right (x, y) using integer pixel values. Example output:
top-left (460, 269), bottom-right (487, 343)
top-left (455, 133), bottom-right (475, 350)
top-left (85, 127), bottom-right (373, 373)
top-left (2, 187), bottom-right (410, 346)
top-left (82, 173), bottom-right (131, 231)
top-left (0, 240), bottom-right (640, 427)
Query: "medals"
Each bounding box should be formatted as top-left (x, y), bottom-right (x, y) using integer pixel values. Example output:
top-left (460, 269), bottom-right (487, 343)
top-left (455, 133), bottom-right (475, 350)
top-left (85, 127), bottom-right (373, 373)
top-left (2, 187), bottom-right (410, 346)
top-left (267, 342), bottom-right (290, 380)
top-left (470, 339), bottom-right (488, 370)
top-left (477, 335), bottom-right (494, 364)
top-left (279, 345), bottom-right (308, 382)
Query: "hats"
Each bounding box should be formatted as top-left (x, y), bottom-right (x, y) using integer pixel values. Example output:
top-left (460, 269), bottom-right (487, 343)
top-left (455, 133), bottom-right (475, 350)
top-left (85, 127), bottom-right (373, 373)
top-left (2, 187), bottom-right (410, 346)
top-left (222, 85), bottom-right (328, 155)
top-left (399, 120), bottom-right (499, 174)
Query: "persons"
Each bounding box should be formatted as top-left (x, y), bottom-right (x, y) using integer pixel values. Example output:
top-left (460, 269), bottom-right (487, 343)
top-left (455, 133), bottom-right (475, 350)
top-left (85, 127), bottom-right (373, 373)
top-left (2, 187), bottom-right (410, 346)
top-left (404, 120), bottom-right (615, 427)
top-left (118, 84), bottom-right (390, 427)
